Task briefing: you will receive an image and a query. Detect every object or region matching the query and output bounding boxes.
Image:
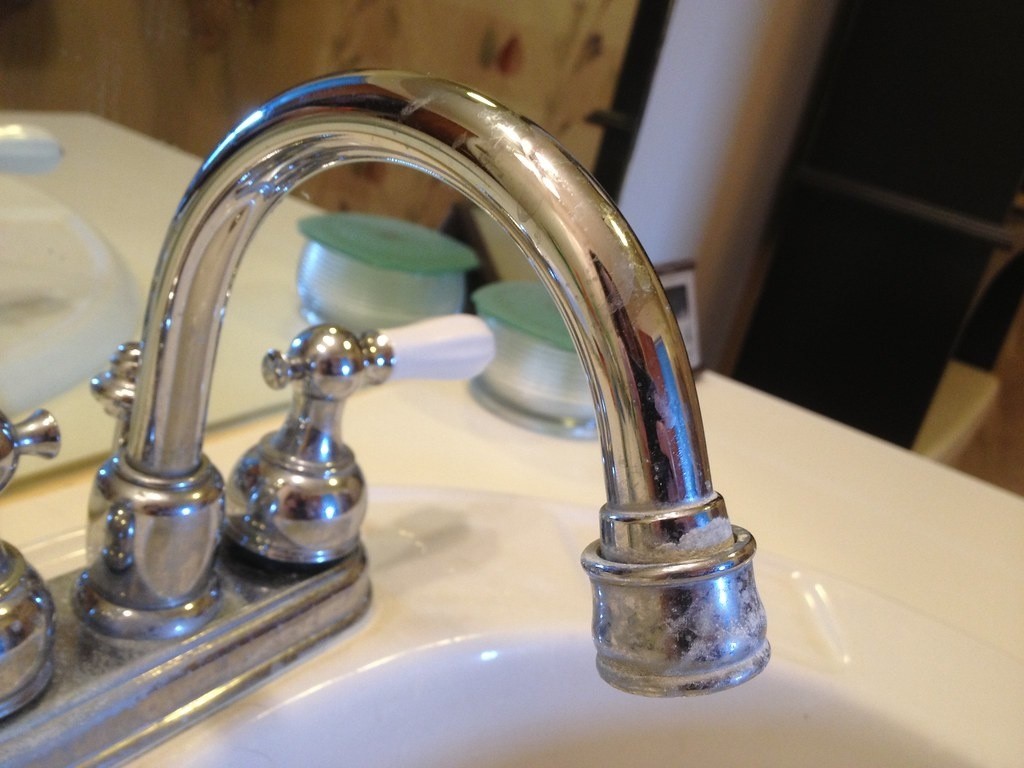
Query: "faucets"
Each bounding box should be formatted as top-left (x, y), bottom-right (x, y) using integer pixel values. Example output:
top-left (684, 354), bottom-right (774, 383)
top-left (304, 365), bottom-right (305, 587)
top-left (68, 66), bottom-right (772, 699)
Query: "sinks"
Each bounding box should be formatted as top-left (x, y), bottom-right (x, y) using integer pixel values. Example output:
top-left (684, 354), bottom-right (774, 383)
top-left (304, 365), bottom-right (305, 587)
top-left (167, 618), bottom-right (978, 768)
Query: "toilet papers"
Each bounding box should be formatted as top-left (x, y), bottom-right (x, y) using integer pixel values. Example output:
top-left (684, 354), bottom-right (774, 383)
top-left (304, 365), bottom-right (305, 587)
top-left (608, 1), bottom-right (835, 373)
